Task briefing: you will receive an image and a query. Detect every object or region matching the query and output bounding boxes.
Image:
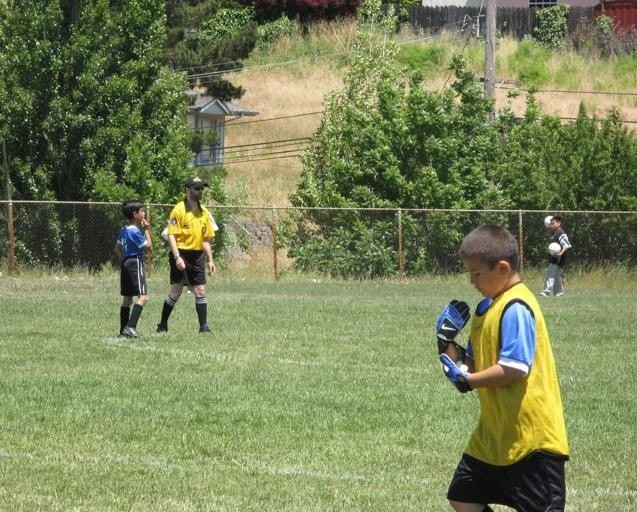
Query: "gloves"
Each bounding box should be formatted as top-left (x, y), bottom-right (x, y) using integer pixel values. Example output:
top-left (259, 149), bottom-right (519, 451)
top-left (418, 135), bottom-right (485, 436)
top-left (437, 299), bottom-right (472, 394)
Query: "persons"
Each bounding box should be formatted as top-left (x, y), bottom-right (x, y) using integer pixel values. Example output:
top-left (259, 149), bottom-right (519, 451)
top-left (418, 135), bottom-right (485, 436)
top-left (112, 200), bottom-right (153, 339)
top-left (154, 175), bottom-right (220, 333)
top-left (538, 216), bottom-right (573, 298)
top-left (433, 223), bottom-right (571, 512)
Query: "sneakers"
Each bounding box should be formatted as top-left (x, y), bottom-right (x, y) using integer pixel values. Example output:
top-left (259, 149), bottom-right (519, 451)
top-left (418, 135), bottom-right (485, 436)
top-left (199, 324), bottom-right (211, 333)
top-left (539, 292), bottom-right (564, 297)
top-left (156, 319), bottom-right (168, 334)
top-left (121, 326), bottom-right (140, 337)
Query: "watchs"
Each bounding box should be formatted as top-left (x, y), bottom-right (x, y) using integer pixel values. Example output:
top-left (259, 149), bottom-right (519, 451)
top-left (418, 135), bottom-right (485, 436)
top-left (173, 254), bottom-right (181, 260)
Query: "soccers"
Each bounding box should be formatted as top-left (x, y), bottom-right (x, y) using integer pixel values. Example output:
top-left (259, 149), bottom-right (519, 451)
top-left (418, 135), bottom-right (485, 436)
top-left (544, 215), bottom-right (555, 228)
top-left (549, 242), bottom-right (562, 257)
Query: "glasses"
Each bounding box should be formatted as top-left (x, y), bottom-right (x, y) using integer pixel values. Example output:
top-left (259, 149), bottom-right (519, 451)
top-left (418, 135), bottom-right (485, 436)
top-left (196, 186), bottom-right (205, 191)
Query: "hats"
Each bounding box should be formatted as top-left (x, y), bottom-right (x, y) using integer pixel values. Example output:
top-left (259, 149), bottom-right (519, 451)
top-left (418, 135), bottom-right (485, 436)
top-left (185, 177), bottom-right (208, 188)
